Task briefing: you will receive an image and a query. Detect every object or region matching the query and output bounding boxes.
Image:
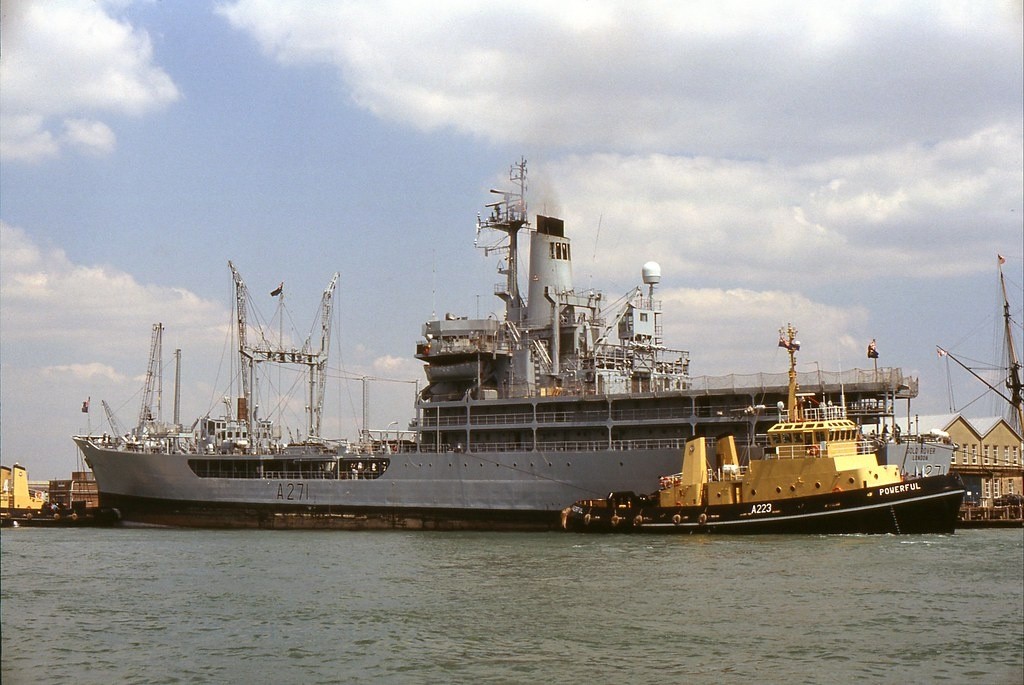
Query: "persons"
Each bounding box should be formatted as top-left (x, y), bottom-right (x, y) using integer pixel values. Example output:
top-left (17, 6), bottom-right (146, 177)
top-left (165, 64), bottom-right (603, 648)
top-left (108, 435), bottom-right (111, 443)
top-left (870, 424), bottom-right (900, 439)
top-left (349, 461), bottom-right (382, 471)
top-left (103, 432), bottom-right (107, 443)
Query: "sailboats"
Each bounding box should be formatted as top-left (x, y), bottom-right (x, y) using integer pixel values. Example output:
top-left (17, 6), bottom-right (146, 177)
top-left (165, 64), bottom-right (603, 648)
top-left (936, 255), bottom-right (1023, 430)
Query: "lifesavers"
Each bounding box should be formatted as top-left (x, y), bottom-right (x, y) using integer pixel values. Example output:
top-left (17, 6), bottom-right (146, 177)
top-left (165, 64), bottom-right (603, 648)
top-left (584, 513), bottom-right (591, 525)
top-left (698, 513), bottom-right (708, 527)
top-left (632, 515), bottom-right (644, 528)
top-left (831, 486), bottom-right (842, 493)
top-left (610, 514), bottom-right (620, 527)
top-left (809, 444), bottom-right (819, 456)
top-left (71, 513), bottom-right (78, 521)
top-left (672, 514), bottom-right (681, 524)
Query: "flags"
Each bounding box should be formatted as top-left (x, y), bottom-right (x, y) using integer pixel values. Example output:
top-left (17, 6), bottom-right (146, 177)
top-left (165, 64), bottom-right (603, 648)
top-left (82, 401), bottom-right (89, 412)
top-left (271, 283), bottom-right (282, 296)
top-left (868, 341), bottom-right (879, 359)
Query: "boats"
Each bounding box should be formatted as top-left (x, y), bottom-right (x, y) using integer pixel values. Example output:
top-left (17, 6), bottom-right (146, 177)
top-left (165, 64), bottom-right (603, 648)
top-left (70, 160), bottom-right (959, 529)
top-left (559, 323), bottom-right (966, 535)
